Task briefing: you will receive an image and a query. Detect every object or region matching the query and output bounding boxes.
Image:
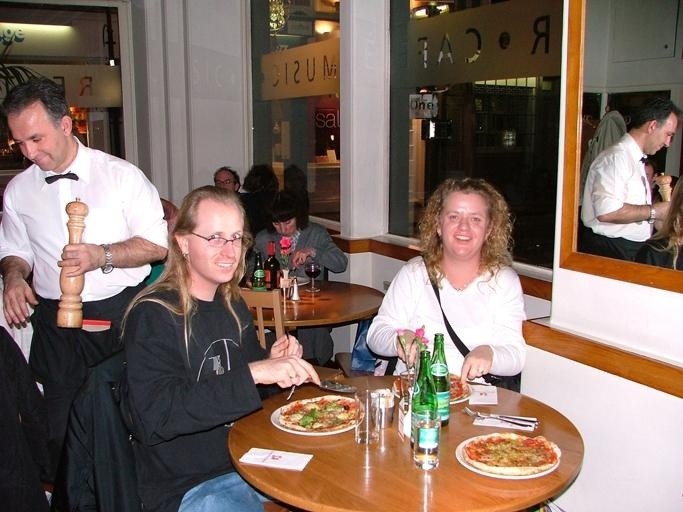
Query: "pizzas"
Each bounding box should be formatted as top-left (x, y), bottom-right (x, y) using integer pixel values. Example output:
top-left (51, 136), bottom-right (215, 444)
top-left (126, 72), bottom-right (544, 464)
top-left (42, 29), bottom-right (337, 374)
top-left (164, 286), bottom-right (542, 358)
top-left (277, 395), bottom-right (362, 432)
top-left (462, 429), bottom-right (561, 476)
top-left (392, 371), bottom-right (471, 403)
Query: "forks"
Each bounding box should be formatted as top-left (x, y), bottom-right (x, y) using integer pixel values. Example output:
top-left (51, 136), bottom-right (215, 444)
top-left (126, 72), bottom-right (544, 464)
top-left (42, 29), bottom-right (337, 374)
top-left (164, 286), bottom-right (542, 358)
top-left (463, 407), bottom-right (532, 428)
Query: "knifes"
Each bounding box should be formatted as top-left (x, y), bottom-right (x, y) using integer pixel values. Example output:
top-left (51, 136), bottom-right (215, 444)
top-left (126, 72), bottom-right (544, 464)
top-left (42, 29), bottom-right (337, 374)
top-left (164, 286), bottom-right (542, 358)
top-left (476, 410), bottom-right (537, 425)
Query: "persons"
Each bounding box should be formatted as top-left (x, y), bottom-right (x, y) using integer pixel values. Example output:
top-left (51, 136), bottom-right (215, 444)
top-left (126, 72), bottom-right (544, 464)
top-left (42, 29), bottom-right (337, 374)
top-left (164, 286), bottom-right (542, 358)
top-left (0, 323), bottom-right (63, 512)
top-left (366, 177), bottom-right (527, 394)
top-left (576, 97), bottom-right (683, 273)
top-left (117, 183), bottom-right (325, 511)
top-left (283, 163), bottom-right (310, 230)
top-left (243, 163), bottom-right (278, 235)
top-left (244, 189), bottom-right (349, 369)
top-left (145, 196), bottom-right (181, 286)
top-left (1, 72), bottom-right (169, 483)
top-left (213, 164), bottom-right (242, 193)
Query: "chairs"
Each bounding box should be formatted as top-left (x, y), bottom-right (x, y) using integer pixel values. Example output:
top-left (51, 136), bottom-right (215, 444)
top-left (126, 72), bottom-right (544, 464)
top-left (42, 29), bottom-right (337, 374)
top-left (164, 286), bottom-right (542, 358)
top-left (231, 287), bottom-right (342, 396)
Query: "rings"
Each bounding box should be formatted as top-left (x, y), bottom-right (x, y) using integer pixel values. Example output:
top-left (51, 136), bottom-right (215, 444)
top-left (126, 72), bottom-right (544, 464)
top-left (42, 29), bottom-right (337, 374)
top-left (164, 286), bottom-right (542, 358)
top-left (477, 366), bottom-right (488, 374)
top-left (290, 374), bottom-right (299, 379)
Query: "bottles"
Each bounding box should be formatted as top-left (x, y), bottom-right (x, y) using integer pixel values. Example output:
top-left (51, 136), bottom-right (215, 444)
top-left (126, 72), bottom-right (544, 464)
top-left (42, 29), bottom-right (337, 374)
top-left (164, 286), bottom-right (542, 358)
top-left (428, 334), bottom-right (449, 425)
top-left (414, 350), bottom-right (437, 447)
top-left (253, 253), bottom-right (265, 291)
top-left (265, 241), bottom-right (279, 290)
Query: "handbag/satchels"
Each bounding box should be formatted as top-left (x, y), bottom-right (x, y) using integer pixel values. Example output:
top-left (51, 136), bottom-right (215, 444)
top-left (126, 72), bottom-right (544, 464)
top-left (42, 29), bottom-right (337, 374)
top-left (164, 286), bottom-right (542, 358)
top-left (487, 371), bottom-right (520, 394)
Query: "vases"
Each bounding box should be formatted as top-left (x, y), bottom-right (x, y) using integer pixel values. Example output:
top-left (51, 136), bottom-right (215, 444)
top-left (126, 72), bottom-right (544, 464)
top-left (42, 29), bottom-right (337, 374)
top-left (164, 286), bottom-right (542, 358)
top-left (280, 268), bottom-right (294, 300)
top-left (400, 370), bottom-right (418, 399)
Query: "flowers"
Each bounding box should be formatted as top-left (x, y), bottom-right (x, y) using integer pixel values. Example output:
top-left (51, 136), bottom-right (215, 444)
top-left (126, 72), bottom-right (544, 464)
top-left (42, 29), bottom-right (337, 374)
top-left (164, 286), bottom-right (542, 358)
top-left (279, 237), bottom-right (293, 271)
top-left (396, 326), bottom-right (429, 388)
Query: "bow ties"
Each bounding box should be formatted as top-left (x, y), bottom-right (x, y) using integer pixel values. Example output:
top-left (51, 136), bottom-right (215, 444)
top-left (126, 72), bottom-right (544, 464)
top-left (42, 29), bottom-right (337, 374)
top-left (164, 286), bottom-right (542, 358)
top-left (45, 173), bottom-right (79, 185)
top-left (640, 157), bottom-right (648, 164)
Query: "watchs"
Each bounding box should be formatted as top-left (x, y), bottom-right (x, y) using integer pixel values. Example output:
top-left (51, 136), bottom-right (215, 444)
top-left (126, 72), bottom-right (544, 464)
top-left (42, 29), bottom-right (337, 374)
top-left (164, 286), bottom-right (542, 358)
top-left (99, 244), bottom-right (115, 275)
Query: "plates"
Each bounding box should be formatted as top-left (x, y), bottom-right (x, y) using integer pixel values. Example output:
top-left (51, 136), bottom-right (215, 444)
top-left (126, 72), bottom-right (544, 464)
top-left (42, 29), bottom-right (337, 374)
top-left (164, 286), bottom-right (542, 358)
top-left (454, 436), bottom-right (560, 480)
top-left (391, 375), bottom-right (472, 405)
top-left (269, 396), bottom-right (364, 437)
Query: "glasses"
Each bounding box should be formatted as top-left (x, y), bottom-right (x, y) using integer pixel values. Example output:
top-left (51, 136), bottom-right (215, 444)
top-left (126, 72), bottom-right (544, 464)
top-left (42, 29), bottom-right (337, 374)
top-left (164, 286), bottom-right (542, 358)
top-left (215, 179), bottom-right (233, 185)
top-left (189, 231), bottom-right (252, 249)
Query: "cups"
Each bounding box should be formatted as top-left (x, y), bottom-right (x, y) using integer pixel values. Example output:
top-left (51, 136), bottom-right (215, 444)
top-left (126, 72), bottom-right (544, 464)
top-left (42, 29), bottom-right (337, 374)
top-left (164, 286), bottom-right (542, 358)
top-left (376, 393), bottom-right (393, 428)
top-left (399, 372), bottom-right (418, 395)
top-left (414, 412), bottom-right (437, 468)
top-left (353, 392), bottom-right (379, 444)
top-left (279, 279), bottom-right (293, 299)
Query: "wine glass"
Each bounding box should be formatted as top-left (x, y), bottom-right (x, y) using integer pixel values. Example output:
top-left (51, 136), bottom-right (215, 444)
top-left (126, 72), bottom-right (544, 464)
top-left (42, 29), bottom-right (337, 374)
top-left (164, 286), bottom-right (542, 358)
top-left (306, 262), bottom-right (321, 292)
